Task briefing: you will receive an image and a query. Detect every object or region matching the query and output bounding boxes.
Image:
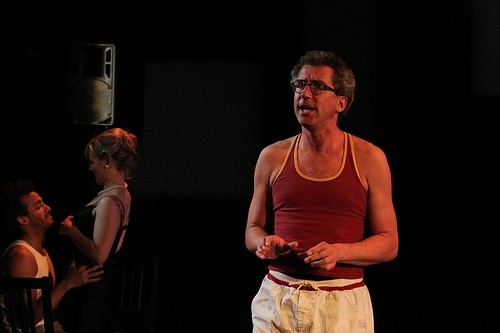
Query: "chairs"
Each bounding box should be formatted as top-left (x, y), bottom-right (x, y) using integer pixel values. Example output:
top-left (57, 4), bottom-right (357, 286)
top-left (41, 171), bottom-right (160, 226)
top-left (0, 276), bottom-right (54, 333)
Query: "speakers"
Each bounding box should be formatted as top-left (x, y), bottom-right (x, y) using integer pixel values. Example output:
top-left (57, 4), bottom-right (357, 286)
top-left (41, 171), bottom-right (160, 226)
top-left (68, 44), bottom-right (115, 126)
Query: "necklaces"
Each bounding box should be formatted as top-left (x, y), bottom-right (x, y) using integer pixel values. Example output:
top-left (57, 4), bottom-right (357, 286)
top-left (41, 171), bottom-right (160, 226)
top-left (98, 182), bottom-right (129, 195)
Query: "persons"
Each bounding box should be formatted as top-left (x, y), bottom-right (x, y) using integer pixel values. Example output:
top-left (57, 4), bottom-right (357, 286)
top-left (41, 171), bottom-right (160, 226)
top-left (60, 128), bottom-right (137, 333)
top-left (0, 181), bottom-right (104, 333)
top-left (245, 51), bottom-right (399, 333)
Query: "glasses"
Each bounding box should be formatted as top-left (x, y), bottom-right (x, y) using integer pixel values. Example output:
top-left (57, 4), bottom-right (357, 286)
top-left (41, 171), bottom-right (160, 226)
top-left (290, 80), bottom-right (338, 95)
